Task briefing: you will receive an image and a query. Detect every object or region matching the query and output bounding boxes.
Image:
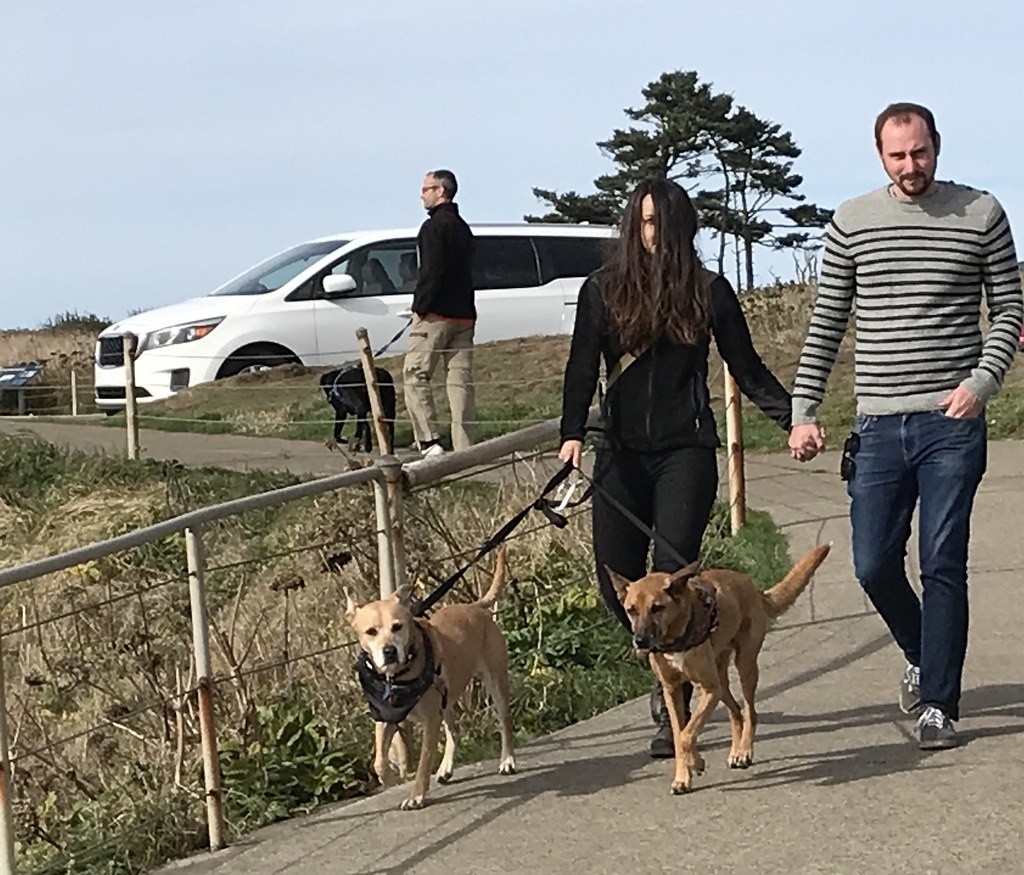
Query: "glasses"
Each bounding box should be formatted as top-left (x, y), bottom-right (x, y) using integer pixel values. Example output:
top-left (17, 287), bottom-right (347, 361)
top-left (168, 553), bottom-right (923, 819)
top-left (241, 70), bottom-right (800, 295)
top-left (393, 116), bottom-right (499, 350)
top-left (840, 430), bottom-right (860, 482)
top-left (422, 185), bottom-right (440, 195)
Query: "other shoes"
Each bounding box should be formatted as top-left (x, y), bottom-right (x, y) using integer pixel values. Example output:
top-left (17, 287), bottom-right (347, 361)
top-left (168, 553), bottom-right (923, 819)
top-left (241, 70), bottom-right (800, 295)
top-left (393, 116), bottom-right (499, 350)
top-left (421, 442), bottom-right (445, 459)
top-left (649, 704), bottom-right (692, 758)
top-left (649, 675), bottom-right (664, 721)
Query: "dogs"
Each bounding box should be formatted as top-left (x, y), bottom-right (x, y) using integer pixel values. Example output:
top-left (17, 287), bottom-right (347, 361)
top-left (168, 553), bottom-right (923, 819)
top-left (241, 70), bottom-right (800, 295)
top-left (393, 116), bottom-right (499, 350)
top-left (342, 542), bottom-right (518, 811)
top-left (318, 366), bottom-right (397, 455)
top-left (604, 544), bottom-right (832, 796)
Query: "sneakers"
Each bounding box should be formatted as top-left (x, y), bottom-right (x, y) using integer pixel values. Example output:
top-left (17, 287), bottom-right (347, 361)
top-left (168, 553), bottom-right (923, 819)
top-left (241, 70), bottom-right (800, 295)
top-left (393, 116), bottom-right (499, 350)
top-left (911, 706), bottom-right (958, 749)
top-left (898, 662), bottom-right (921, 715)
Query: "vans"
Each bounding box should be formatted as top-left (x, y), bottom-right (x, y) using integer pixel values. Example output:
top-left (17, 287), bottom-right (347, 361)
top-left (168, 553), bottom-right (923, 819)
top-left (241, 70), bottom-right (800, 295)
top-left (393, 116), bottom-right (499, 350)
top-left (91, 221), bottom-right (621, 419)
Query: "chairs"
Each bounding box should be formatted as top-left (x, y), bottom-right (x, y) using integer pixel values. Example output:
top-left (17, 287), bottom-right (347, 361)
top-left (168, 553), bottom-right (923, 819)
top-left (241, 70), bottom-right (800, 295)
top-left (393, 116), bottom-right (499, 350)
top-left (400, 253), bottom-right (418, 294)
top-left (362, 258), bottom-right (397, 295)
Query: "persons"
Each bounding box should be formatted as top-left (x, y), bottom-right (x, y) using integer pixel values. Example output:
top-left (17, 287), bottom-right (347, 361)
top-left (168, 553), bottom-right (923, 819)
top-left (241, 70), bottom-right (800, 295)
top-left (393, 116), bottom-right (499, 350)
top-left (790, 103), bottom-right (1024, 750)
top-left (560, 178), bottom-right (825, 756)
top-left (402, 171), bottom-right (477, 460)
